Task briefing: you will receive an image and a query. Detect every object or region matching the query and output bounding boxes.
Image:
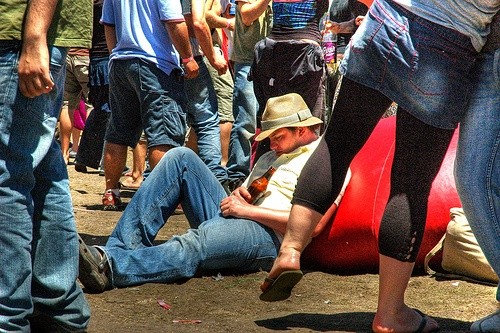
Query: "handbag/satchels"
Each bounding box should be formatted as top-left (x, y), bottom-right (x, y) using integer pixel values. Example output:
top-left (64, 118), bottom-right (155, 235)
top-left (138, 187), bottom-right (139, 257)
top-left (423, 206), bottom-right (500, 282)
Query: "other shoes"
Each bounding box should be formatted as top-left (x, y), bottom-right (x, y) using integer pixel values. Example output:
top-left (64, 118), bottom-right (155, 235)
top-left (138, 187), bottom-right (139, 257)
top-left (221, 180), bottom-right (230, 197)
top-left (471, 309), bottom-right (500, 333)
top-left (68, 148), bottom-right (78, 158)
top-left (76, 161), bottom-right (87, 173)
top-left (143, 161), bottom-right (151, 177)
top-left (100, 168), bottom-right (130, 175)
top-left (230, 177), bottom-right (246, 192)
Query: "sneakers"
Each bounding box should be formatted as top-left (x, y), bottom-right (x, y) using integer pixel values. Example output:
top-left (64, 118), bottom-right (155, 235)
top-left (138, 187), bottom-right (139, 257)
top-left (102, 189), bottom-right (122, 210)
top-left (173, 204), bottom-right (184, 214)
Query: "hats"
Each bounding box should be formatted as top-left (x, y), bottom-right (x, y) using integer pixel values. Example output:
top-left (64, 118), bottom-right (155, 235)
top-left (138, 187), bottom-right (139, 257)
top-left (254, 92), bottom-right (324, 142)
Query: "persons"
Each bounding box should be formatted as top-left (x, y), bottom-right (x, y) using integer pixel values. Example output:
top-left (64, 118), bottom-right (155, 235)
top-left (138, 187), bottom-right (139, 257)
top-left (0, 0), bottom-right (95, 333)
top-left (56, 0), bottom-right (374, 211)
top-left (261, 0), bottom-right (500, 333)
top-left (75, 94), bottom-right (350, 295)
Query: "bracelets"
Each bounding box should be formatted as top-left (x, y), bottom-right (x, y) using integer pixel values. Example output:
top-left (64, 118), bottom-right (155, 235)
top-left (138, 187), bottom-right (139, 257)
top-left (226, 19), bottom-right (230, 29)
top-left (182, 55), bottom-right (193, 64)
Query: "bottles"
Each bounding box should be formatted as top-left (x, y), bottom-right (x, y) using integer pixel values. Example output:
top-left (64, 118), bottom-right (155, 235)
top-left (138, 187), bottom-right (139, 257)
top-left (322, 23), bottom-right (338, 65)
top-left (228, 0), bottom-right (236, 16)
top-left (246, 166), bottom-right (276, 204)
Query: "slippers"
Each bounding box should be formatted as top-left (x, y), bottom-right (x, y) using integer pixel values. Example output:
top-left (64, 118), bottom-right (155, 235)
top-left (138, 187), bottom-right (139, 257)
top-left (119, 182), bottom-right (139, 190)
top-left (76, 231), bottom-right (109, 294)
top-left (413, 309), bottom-right (442, 333)
top-left (259, 269), bottom-right (305, 303)
